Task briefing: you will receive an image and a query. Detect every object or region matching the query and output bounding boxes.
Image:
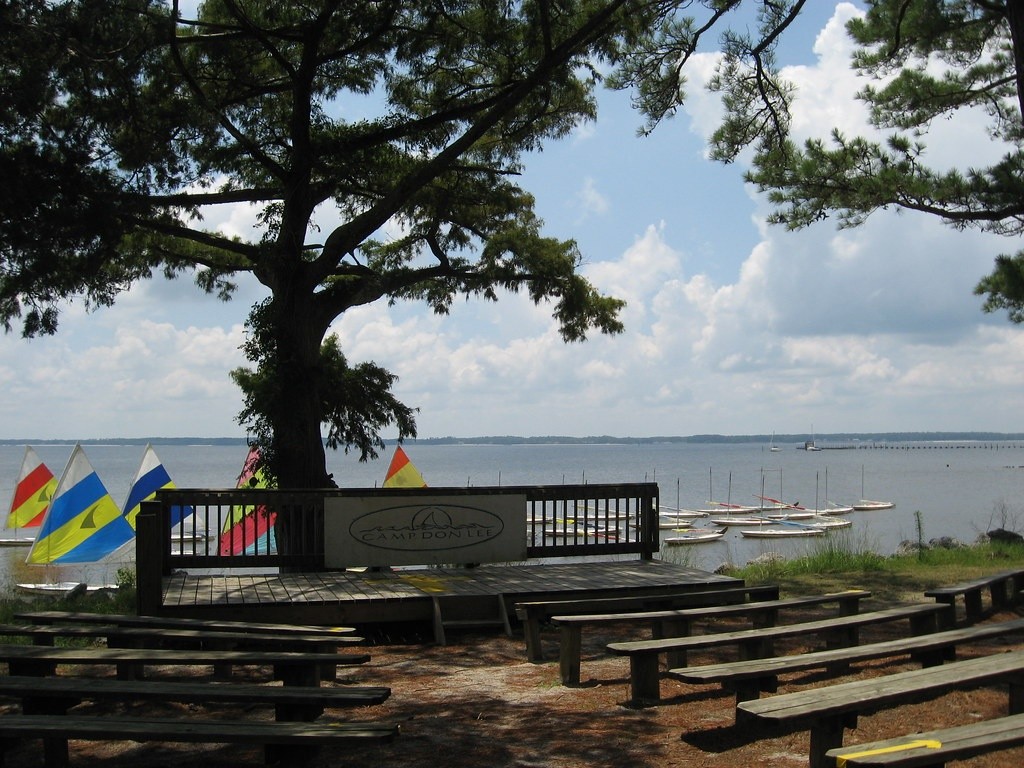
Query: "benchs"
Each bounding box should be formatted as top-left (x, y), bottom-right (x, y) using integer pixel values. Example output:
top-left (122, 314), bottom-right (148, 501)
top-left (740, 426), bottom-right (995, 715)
top-left (0, 678), bottom-right (392, 722)
top-left (514, 585), bottom-right (779, 663)
top-left (13, 611), bottom-right (356, 681)
top-left (606, 604), bottom-right (951, 704)
top-left (0, 623), bottom-right (366, 681)
top-left (668, 618), bottom-right (1024, 706)
top-left (924, 570), bottom-right (1024, 626)
top-left (551, 590), bottom-right (871, 687)
top-left (0, 715), bottom-right (402, 768)
top-left (0, 633), bottom-right (371, 687)
top-left (825, 713), bottom-right (1024, 768)
top-left (735, 650), bottom-right (1024, 768)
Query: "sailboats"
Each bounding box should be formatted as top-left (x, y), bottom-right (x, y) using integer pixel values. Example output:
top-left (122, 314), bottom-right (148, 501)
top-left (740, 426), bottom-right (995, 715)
top-left (0, 445), bottom-right (59, 546)
top-left (526, 464), bottom-right (894, 544)
top-left (122, 441), bottom-right (211, 541)
top-left (16, 441), bottom-right (137, 595)
top-left (770, 431), bottom-right (781, 451)
top-left (807, 424), bottom-right (822, 450)
